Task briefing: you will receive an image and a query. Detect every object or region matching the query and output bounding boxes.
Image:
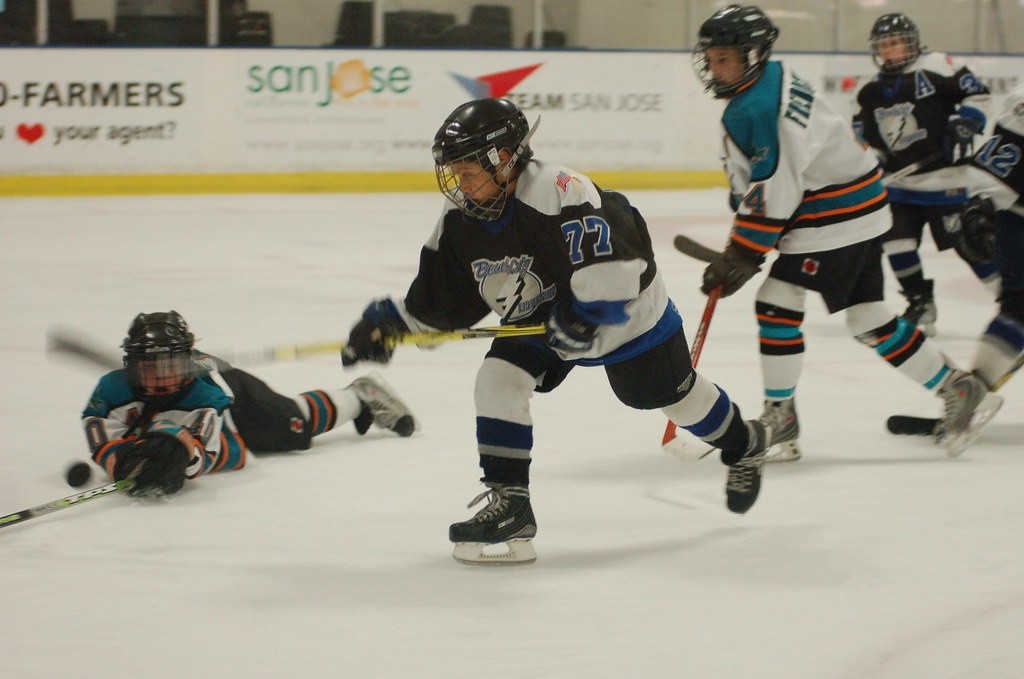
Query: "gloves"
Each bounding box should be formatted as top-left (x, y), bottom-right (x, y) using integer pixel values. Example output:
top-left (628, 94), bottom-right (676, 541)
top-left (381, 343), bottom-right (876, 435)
top-left (114, 446), bottom-right (184, 498)
top-left (340, 316), bottom-right (397, 368)
top-left (700, 247), bottom-right (764, 298)
top-left (545, 312), bottom-right (600, 354)
top-left (946, 120), bottom-right (972, 145)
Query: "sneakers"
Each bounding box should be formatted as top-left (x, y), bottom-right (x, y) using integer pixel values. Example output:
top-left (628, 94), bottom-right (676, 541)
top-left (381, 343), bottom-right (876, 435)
top-left (449, 481), bottom-right (538, 567)
top-left (755, 398), bottom-right (803, 463)
top-left (725, 419), bottom-right (773, 515)
top-left (901, 279), bottom-right (936, 336)
top-left (346, 369), bottom-right (421, 437)
top-left (933, 370), bottom-right (1002, 456)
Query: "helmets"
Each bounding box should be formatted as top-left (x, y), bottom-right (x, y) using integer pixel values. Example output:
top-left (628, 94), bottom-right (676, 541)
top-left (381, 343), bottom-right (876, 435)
top-left (691, 4), bottom-right (779, 100)
top-left (120, 311), bottom-right (197, 402)
top-left (432, 98), bottom-right (541, 222)
top-left (869, 14), bottom-right (921, 74)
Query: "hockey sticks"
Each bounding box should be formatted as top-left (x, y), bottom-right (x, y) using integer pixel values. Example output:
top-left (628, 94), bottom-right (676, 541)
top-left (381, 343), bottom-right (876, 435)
top-left (41, 320), bottom-right (549, 379)
top-left (658, 283), bottom-right (725, 463)
top-left (0, 476), bottom-right (138, 530)
top-left (882, 136), bottom-right (958, 187)
top-left (675, 234), bottom-right (727, 264)
top-left (887, 413), bottom-right (943, 437)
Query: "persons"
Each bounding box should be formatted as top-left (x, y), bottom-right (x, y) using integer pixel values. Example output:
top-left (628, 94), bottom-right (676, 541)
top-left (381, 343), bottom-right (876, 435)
top-left (80, 311), bottom-right (415, 497)
top-left (341, 98), bottom-right (773, 566)
top-left (700, 5), bottom-right (1003, 463)
top-left (852, 13), bottom-right (1024, 394)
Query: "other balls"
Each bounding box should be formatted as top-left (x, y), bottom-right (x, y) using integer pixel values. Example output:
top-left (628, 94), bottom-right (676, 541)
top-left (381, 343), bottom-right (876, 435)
top-left (64, 462), bottom-right (92, 488)
